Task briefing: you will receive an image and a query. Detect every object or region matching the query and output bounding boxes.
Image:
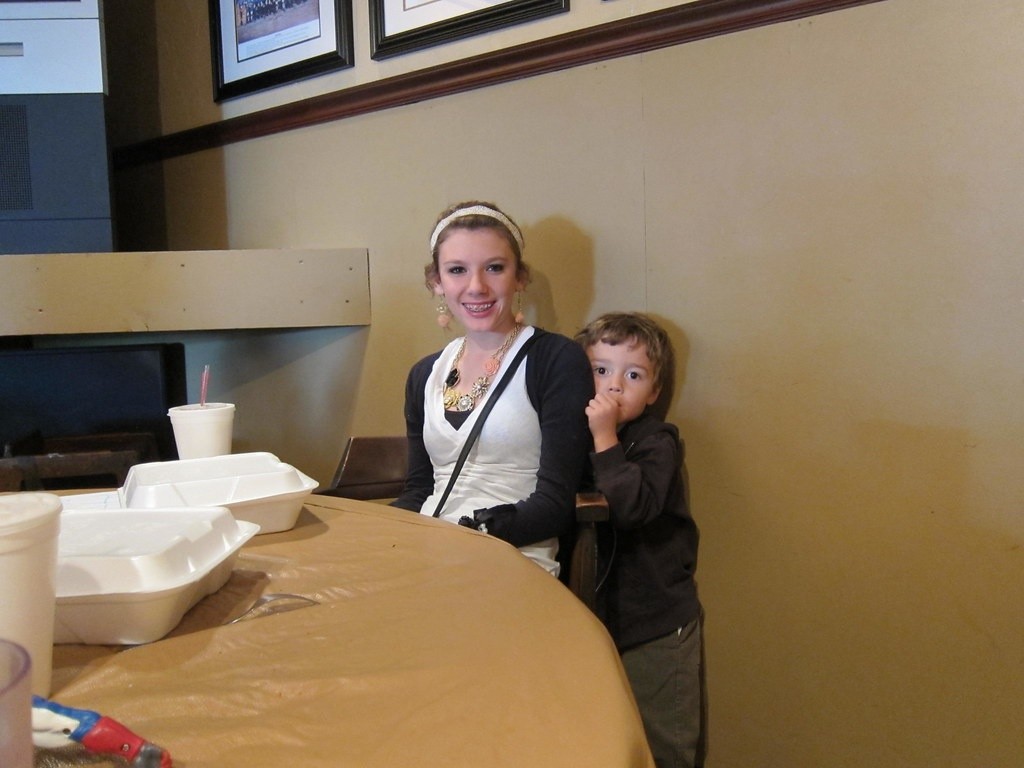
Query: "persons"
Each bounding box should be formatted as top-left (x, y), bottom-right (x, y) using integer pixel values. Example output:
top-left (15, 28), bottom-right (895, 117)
top-left (386, 199), bottom-right (598, 579)
top-left (577, 307), bottom-right (708, 767)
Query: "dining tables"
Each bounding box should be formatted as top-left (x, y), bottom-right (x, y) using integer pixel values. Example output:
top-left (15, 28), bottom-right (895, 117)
top-left (0, 488), bottom-right (654, 768)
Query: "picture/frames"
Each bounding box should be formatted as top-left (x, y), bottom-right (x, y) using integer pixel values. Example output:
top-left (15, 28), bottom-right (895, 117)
top-left (208, 0), bottom-right (354, 103)
top-left (368, 1), bottom-right (570, 61)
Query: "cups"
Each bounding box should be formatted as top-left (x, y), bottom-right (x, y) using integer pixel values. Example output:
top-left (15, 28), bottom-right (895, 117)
top-left (0, 639), bottom-right (34, 768)
top-left (1, 494), bottom-right (62, 699)
top-left (167, 403), bottom-right (236, 461)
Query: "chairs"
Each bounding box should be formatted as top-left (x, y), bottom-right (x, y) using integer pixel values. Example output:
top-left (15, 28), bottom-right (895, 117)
top-left (311, 436), bottom-right (610, 613)
top-left (0, 451), bottom-right (141, 491)
top-left (42, 433), bottom-right (161, 464)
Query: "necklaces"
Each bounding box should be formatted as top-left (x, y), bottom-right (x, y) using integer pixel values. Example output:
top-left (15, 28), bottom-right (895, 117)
top-left (442, 323), bottom-right (521, 414)
